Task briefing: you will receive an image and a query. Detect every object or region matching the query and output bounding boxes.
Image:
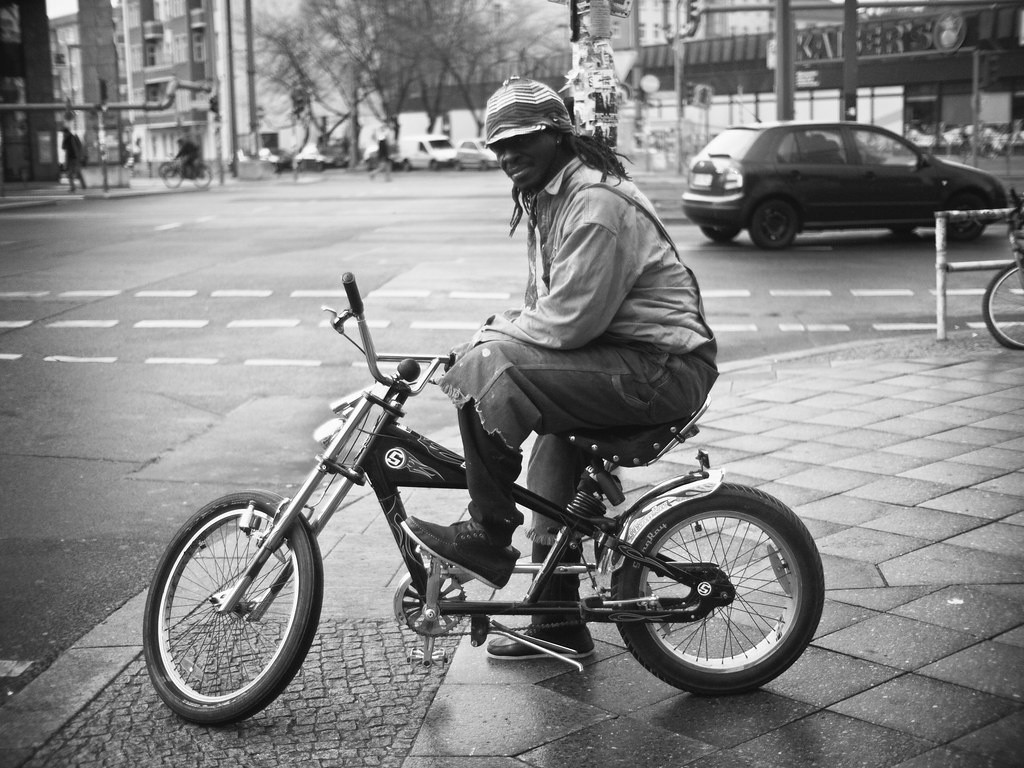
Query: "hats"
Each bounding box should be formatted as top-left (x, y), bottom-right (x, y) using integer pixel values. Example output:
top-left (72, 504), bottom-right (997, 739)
top-left (484, 76), bottom-right (575, 149)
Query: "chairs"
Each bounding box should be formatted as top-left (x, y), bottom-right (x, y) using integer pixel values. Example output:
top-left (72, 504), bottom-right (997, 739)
top-left (791, 134), bottom-right (846, 165)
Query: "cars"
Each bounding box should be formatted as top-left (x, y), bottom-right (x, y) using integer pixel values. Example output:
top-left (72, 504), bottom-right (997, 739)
top-left (682, 122), bottom-right (1007, 253)
top-left (457, 138), bottom-right (504, 171)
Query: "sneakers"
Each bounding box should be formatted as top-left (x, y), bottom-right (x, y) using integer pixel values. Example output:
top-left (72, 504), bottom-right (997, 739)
top-left (486, 624), bottom-right (595, 660)
top-left (401, 516), bottom-right (521, 589)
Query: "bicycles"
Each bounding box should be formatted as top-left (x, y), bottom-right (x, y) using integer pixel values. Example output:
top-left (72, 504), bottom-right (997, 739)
top-left (143, 271), bottom-right (825, 729)
top-left (982, 185), bottom-right (1024, 353)
top-left (157, 155), bottom-right (210, 190)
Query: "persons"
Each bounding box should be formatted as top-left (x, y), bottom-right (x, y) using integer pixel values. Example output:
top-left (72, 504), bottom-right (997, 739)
top-left (395, 72), bottom-right (723, 661)
top-left (173, 138), bottom-right (200, 179)
top-left (62, 127), bottom-right (86, 193)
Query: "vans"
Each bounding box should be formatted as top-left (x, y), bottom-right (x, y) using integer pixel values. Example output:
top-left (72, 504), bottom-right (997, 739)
top-left (391, 134), bottom-right (459, 171)
